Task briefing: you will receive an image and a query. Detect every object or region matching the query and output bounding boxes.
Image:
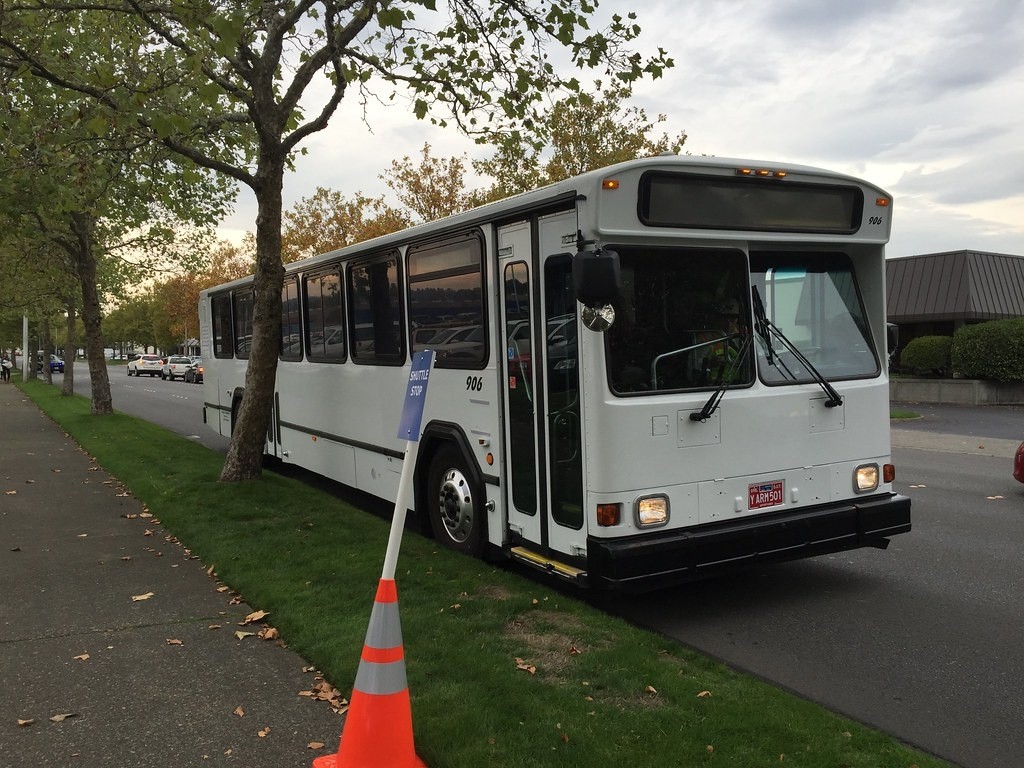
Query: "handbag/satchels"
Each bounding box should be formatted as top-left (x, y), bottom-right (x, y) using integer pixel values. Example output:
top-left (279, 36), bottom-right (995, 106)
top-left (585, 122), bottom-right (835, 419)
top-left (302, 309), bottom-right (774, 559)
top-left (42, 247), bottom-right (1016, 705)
top-left (2, 360), bottom-right (13, 369)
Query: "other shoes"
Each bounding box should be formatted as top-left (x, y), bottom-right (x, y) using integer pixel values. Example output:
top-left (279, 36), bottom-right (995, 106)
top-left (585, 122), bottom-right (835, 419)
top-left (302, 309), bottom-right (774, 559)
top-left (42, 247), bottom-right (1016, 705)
top-left (4, 380), bottom-right (10, 384)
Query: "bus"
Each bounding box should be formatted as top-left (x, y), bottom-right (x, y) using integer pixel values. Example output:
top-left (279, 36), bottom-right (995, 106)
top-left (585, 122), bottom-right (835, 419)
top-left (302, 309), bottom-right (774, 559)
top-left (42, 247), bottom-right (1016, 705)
top-left (196, 157), bottom-right (913, 607)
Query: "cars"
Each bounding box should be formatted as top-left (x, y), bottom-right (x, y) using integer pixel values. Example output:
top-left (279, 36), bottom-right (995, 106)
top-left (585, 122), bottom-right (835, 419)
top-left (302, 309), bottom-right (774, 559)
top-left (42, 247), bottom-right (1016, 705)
top-left (231, 311), bottom-right (578, 367)
top-left (184, 361), bottom-right (204, 383)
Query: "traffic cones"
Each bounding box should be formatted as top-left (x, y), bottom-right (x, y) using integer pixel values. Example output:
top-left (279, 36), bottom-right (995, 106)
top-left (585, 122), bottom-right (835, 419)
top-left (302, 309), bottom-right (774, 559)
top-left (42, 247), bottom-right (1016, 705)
top-left (309, 578), bottom-right (431, 767)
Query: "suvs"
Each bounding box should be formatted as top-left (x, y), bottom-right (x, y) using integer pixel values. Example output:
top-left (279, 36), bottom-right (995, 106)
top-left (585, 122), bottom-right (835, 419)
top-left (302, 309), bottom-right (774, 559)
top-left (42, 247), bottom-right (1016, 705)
top-left (30, 354), bottom-right (65, 374)
top-left (126, 354), bottom-right (163, 378)
top-left (161, 357), bottom-right (191, 381)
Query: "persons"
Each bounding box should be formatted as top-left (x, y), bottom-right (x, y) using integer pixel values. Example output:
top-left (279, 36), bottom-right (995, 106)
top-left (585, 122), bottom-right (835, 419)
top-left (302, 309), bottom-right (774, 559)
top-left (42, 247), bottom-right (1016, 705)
top-left (0, 351), bottom-right (13, 384)
top-left (689, 296), bottom-right (767, 386)
